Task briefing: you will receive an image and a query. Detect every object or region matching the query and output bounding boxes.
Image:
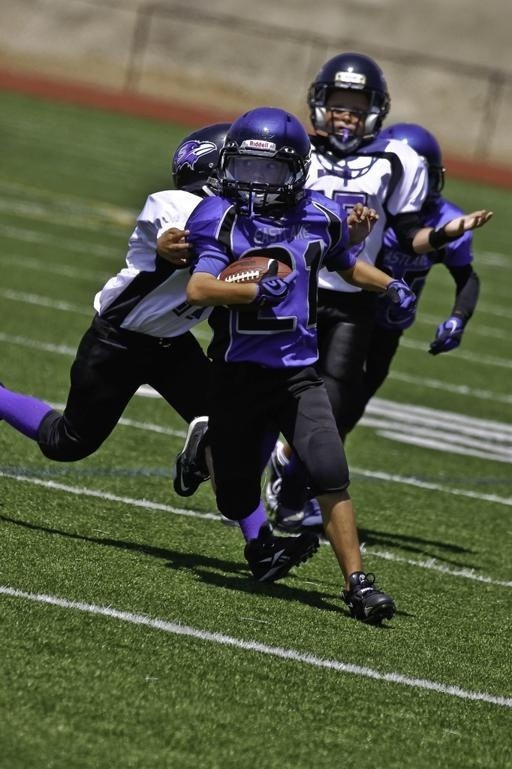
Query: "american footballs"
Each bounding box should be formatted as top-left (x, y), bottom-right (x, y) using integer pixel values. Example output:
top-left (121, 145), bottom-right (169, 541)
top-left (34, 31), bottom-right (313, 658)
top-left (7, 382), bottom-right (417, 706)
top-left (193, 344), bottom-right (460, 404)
top-left (217, 256), bottom-right (292, 309)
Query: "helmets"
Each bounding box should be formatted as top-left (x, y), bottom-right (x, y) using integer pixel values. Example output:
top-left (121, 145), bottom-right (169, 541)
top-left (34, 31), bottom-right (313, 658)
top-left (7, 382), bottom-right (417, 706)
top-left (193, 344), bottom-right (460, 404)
top-left (215, 106), bottom-right (314, 197)
top-left (171, 121), bottom-right (233, 194)
top-left (306, 52), bottom-right (391, 136)
top-left (378, 120), bottom-right (442, 192)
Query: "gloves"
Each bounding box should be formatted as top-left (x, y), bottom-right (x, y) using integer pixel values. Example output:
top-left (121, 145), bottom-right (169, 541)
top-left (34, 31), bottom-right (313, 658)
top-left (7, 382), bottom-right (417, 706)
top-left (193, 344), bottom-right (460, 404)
top-left (428, 313), bottom-right (466, 355)
top-left (247, 270), bottom-right (300, 308)
top-left (384, 277), bottom-right (418, 316)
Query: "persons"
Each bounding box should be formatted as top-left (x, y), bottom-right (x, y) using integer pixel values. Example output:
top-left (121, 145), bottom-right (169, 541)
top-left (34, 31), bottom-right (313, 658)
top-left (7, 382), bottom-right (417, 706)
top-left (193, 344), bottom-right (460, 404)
top-left (0, 120), bottom-right (380, 583)
top-left (265, 123), bottom-right (480, 529)
top-left (276, 53), bottom-right (494, 533)
top-left (173, 107), bottom-right (417, 624)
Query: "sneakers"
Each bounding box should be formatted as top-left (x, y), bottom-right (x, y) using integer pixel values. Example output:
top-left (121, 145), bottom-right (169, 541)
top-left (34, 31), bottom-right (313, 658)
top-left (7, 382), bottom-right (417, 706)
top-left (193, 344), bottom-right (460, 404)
top-left (340, 570), bottom-right (395, 623)
top-left (263, 438), bottom-right (323, 526)
top-left (172, 414), bottom-right (212, 498)
top-left (243, 521), bottom-right (321, 584)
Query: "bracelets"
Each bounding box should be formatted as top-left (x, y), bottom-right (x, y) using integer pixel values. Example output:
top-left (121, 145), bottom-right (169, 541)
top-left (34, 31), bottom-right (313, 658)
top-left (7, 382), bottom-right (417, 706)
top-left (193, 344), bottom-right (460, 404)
top-left (429, 219), bottom-right (465, 252)
